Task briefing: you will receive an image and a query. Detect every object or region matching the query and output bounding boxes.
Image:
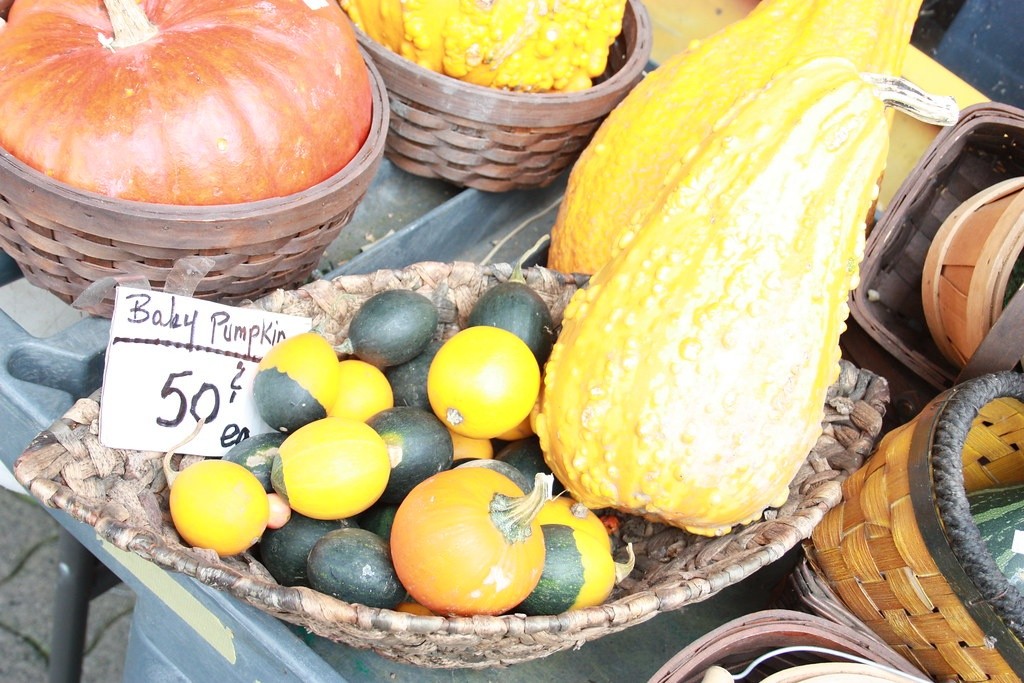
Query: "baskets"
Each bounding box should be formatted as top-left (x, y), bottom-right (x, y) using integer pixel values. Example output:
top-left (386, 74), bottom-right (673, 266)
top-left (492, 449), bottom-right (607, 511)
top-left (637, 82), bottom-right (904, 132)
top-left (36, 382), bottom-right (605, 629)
top-left (1, 43), bottom-right (390, 323)
top-left (3, 254), bottom-right (899, 672)
top-left (343, 0), bottom-right (655, 192)
top-left (801, 365), bottom-right (1022, 682)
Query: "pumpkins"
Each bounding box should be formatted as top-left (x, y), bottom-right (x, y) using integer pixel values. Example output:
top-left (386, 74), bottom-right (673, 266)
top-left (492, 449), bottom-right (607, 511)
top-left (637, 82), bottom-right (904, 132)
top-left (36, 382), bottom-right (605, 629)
top-left (0, 0), bottom-right (1024, 619)
